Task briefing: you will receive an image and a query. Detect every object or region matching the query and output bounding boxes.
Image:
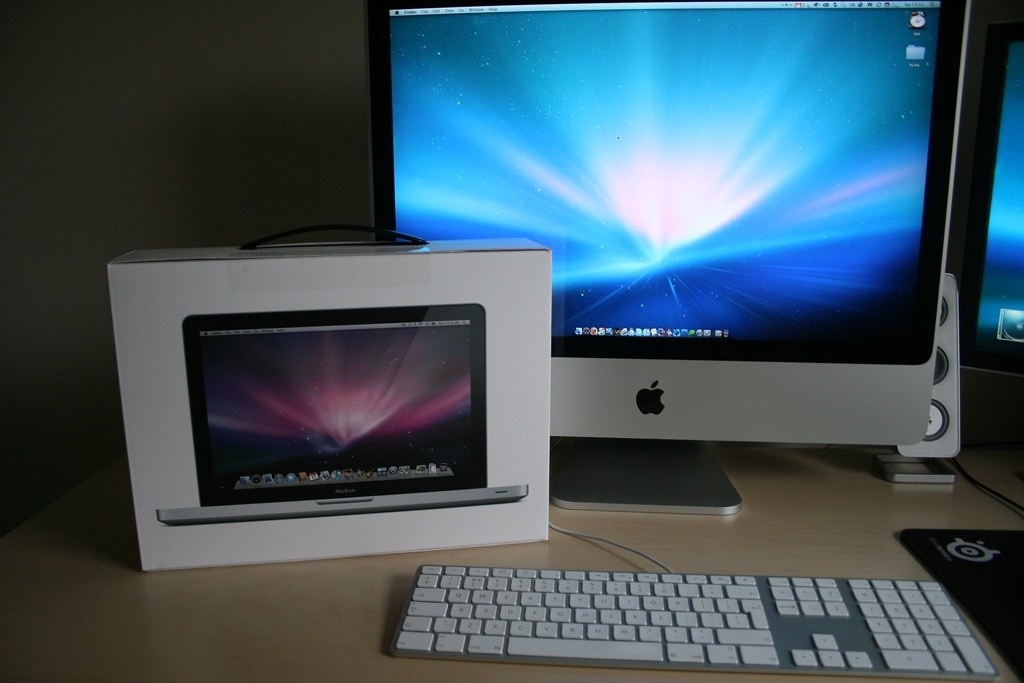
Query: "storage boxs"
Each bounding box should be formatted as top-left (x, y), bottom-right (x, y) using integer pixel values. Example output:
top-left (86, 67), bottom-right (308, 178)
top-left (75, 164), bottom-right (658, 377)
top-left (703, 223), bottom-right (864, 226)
top-left (108, 224), bottom-right (552, 571)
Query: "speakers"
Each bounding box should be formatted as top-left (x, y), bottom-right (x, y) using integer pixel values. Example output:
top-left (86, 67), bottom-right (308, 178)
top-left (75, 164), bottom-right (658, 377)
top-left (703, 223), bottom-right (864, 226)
top-left (873, 273), bottom-right (959, 484)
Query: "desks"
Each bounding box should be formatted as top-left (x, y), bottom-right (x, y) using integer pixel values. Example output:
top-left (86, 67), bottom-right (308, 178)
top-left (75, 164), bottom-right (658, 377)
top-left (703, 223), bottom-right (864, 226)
top-left (0, 446), bottom-right (1024, 683)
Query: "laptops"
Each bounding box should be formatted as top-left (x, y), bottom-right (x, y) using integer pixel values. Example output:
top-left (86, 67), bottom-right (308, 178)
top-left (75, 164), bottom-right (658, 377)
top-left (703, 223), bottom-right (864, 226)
top-left (157, 302), bottom-right (528, 522)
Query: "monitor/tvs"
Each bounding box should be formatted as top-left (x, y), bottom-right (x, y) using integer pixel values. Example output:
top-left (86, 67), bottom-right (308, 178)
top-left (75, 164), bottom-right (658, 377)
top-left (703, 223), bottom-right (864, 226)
top-left (367, 0), bottom-right (972, 515)
top-left (959, 20), bottom-right (1024, 377)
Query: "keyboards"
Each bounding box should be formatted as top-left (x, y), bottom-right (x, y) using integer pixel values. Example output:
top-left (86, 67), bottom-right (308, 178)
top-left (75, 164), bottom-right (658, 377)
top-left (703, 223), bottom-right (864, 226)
top-left (389, 565), bottom-right (1001, 683)
